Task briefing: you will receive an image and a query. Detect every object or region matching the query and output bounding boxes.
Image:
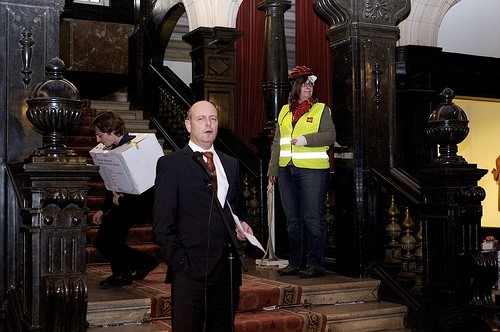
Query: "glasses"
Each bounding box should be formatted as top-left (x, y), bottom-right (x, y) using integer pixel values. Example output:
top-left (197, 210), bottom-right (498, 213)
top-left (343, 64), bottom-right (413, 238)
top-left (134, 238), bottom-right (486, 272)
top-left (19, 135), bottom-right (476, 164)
top-left (301, 82), bottom-right (313, 87)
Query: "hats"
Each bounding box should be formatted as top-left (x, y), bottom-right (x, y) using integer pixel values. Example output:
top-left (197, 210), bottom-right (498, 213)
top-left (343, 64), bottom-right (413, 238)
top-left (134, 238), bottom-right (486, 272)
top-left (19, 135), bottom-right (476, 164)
top-left (287, 65), bottom-right (314, 78)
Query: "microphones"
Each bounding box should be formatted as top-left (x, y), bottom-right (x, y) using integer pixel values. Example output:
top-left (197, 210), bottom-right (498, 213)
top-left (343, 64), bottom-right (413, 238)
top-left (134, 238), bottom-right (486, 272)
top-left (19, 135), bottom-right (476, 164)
top-left (193, 151), bottom-right (215, 180)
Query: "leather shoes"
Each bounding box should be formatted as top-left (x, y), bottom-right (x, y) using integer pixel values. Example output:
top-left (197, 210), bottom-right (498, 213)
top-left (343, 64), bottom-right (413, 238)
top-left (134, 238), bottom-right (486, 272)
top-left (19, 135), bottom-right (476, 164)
top-left (277, 263), bottom-right (302, 275)
top-left (132, 257), bottom-right (159, 280)
top-left (98, 273), bottom-right (132, 288)
top-left (298, 269), bottom-right (327, 279)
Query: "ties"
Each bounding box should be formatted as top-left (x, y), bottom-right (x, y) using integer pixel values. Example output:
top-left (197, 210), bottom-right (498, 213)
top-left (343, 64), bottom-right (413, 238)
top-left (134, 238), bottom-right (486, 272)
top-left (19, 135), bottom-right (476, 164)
top-left (202, 151), bottom-right (218, 197)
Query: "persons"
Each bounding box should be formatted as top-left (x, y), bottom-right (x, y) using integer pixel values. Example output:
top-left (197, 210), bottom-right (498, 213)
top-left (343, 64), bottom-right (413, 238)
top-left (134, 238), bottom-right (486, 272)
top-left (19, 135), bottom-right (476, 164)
top-left (153, 100), bottom-right (253, 332)
top-left (266, 65), bottom-right (337, 278)
top-left (91, 110), bottom-right (165, 290)
